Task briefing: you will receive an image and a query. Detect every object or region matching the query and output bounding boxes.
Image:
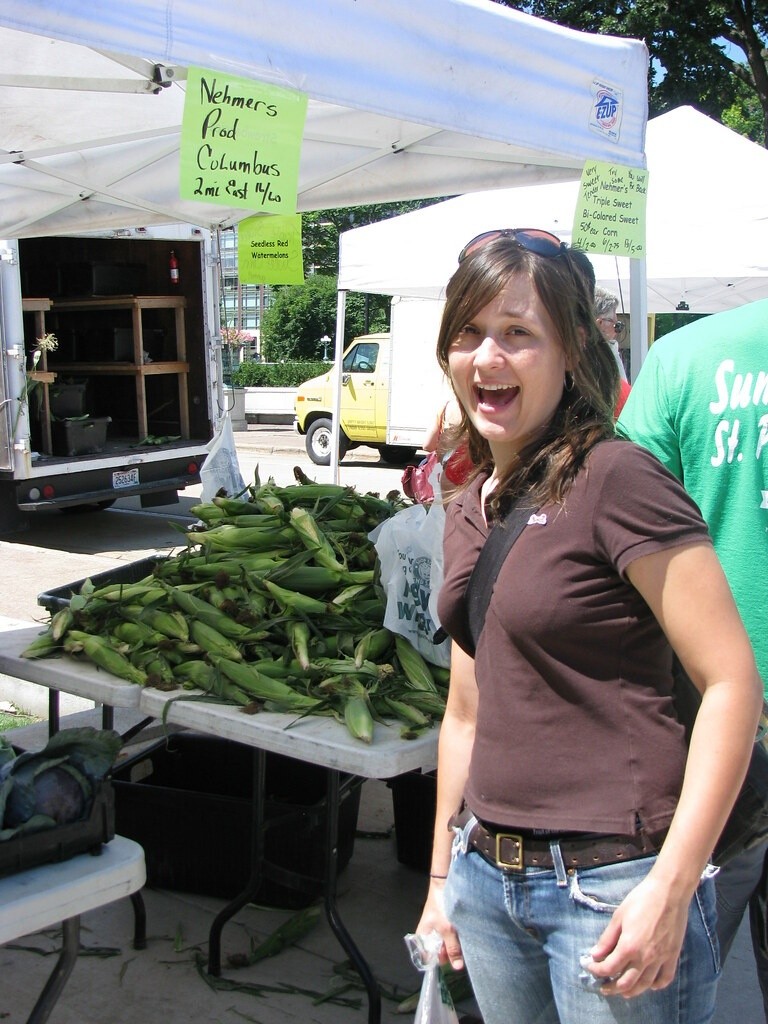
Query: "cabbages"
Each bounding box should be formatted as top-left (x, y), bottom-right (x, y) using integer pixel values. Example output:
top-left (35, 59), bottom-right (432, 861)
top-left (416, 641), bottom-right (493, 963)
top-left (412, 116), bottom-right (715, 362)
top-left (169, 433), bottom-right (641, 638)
top-left (0, 725), bottom-right (123, 843)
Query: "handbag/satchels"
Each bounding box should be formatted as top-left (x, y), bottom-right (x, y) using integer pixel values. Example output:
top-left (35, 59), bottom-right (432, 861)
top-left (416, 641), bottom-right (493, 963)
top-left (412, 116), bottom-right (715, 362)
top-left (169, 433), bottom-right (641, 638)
top-left (369, 465), bottom-right (452, 669)
top-left (404, 931), bottom-right (460, 1024)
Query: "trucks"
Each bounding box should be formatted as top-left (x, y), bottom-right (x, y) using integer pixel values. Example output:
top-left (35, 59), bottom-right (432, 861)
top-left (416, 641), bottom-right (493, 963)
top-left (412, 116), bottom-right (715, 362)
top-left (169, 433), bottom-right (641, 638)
top-left (7, 226), bottom-right (219, 529)
top-left (294, 295), bottom-right (630, 466)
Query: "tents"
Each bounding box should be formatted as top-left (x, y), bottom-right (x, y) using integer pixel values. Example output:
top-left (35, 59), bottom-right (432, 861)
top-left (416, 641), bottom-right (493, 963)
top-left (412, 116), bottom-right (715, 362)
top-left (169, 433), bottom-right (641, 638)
top-left (330, 105), bottom-right (768, 483)
top-left (0, 0), bottom-right (649, 382)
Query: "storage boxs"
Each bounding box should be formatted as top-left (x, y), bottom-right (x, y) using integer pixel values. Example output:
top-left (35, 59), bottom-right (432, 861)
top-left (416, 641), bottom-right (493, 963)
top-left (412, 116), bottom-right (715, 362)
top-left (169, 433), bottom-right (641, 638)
top-left (107, 739), bottom-right (364, 910)
top-left (50, 384), bottom-right (86, 417)
top-left (52, 415), bottom-right (112, 455)
top-left (388, 769), bottom-right (438, 871)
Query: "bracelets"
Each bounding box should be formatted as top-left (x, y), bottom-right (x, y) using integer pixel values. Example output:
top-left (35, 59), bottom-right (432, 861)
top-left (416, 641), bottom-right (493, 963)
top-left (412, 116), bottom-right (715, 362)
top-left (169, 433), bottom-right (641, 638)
top-left (430, 873), bottom-right (447, 879)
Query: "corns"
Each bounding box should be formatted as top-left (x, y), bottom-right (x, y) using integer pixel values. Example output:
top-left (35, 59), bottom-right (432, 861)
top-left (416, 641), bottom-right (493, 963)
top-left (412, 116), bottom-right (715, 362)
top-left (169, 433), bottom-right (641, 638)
top-left (15, 466), bottom-right (452, 742)
top-left (396, 972), bottom-right (475, 1014)
top-left (227, 905), bottom-right (322, 968)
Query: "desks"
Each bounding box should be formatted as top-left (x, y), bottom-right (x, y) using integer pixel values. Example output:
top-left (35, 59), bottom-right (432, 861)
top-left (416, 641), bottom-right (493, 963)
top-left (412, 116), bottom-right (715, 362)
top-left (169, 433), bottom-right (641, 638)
top-left (0, 624), bottom-right (441, 1023)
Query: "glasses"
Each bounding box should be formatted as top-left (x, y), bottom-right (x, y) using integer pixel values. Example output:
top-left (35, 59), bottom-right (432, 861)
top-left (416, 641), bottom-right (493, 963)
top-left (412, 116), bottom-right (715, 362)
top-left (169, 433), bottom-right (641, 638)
top-left (603, 316), bottom-right (624, 332)
top-left (458, 229), bottom-right (578, 304)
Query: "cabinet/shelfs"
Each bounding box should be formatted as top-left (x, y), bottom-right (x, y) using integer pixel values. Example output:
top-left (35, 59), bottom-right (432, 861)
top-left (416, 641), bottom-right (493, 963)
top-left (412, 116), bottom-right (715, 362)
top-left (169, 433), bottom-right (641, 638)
top-left (48, 296), bottom-right (193, 443)
top-left (22, 297), bottom-right (53, 455)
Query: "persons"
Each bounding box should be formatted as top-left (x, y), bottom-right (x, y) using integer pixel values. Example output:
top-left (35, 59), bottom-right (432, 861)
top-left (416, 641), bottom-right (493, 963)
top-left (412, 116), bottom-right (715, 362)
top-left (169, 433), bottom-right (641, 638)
top-left (591, 287), bottom-right (619, 340)
top-left (414, 229), bottom-right (764, 1024)
top-left (613, 298), bottom-right (768, 1015)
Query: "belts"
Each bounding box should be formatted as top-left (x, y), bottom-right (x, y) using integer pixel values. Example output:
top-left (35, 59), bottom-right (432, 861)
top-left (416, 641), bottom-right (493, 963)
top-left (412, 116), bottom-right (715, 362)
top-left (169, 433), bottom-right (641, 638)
top-left (458, 800), bottom-right (672, 869)
